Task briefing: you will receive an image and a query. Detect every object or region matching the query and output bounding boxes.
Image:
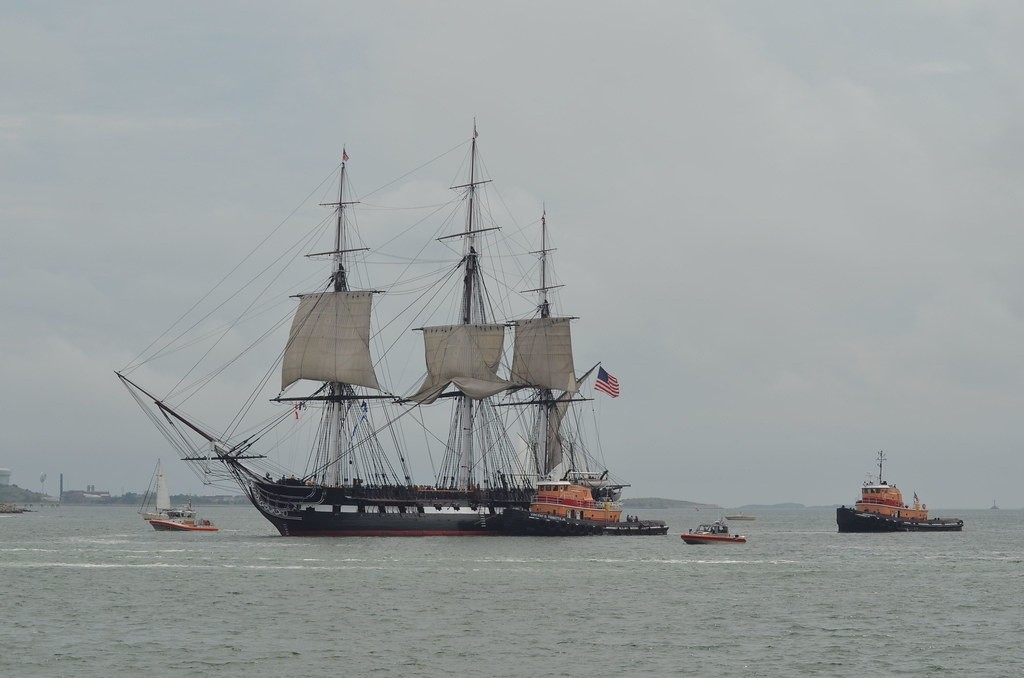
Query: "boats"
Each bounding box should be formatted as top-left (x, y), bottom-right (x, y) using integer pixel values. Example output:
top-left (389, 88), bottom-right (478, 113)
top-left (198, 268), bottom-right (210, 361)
top-left (725, 508), bottom-right (756, 520)
top-left (833, 451), bottom-right (965, 533)
top-left (495, 480), bottom-right (668, 536)
top-left (679, 518), bottom-right (746, 545)
top-left (150, 498), bottom-right (218, 532)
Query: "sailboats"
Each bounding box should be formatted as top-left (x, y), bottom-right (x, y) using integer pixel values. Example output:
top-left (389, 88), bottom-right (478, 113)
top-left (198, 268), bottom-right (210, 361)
top-left (136, 458), bottom-right (199, 522)
top-left (111, 118), bottom-right (631, 535)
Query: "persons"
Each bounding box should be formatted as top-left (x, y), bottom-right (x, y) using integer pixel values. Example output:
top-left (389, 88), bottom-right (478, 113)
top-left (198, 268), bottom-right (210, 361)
top-left (282, 475), bottom-right (285, 479)
top-left (266, 471), bottom-right (269, 478)
top-left (308, 479), bottom-right (312, 486)
top-left (291, 474), bottom-right (295, 479)
top-left (330, 483), bottom-right (458, 490)
top-left (627, 515), bottom-right (638, 523)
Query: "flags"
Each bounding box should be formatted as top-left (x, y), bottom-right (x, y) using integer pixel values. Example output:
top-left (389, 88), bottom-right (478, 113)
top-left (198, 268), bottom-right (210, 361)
top-left (914, 492), bottom-right (919, 501)
top-left (594, 366), bottom-right (620, 399)
